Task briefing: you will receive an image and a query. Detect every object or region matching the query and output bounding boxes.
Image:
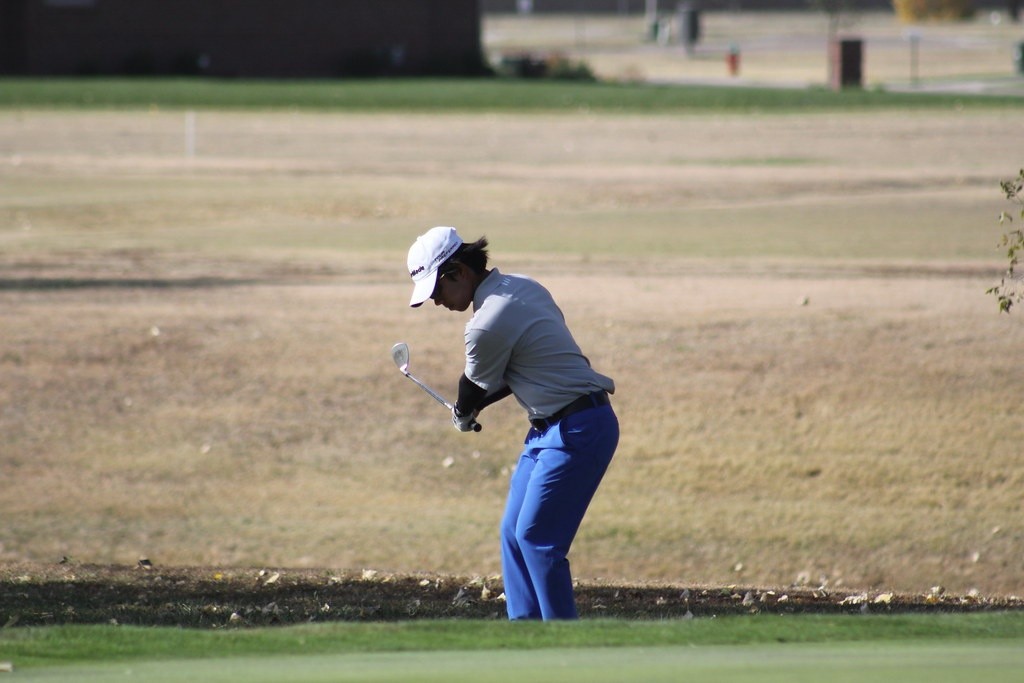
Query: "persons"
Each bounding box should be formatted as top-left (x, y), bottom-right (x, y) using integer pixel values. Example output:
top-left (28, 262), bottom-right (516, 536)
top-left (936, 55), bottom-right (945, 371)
top-left (407, 226), bottom-right (621, 620)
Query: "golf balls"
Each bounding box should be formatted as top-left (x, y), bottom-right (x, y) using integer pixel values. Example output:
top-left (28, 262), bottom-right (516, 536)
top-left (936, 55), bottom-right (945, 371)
top-left (443, 456), bottom-right (456, 469)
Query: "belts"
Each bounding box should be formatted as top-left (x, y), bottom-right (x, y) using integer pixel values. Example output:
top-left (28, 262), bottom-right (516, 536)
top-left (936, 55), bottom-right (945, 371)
top-left (530, 392), bottom-right (610, 434)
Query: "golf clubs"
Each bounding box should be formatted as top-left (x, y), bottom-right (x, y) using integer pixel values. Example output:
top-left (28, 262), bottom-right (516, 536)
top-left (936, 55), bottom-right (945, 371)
top-left (390, 342), bottom-right (482, 433)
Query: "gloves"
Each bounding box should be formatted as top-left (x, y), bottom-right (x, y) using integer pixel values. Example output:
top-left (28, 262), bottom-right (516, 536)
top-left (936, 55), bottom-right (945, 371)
top-left (452, 402), bottom-right (476, 432)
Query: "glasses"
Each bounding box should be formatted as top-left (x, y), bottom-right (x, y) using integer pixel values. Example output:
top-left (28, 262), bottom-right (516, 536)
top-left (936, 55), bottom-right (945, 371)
top-left (430, 257), bottom-right (455, 299)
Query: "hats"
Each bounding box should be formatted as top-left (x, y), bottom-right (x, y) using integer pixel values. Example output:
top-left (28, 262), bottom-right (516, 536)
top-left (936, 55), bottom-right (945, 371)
top-left (407, 227), bottom-right (463, 308)
